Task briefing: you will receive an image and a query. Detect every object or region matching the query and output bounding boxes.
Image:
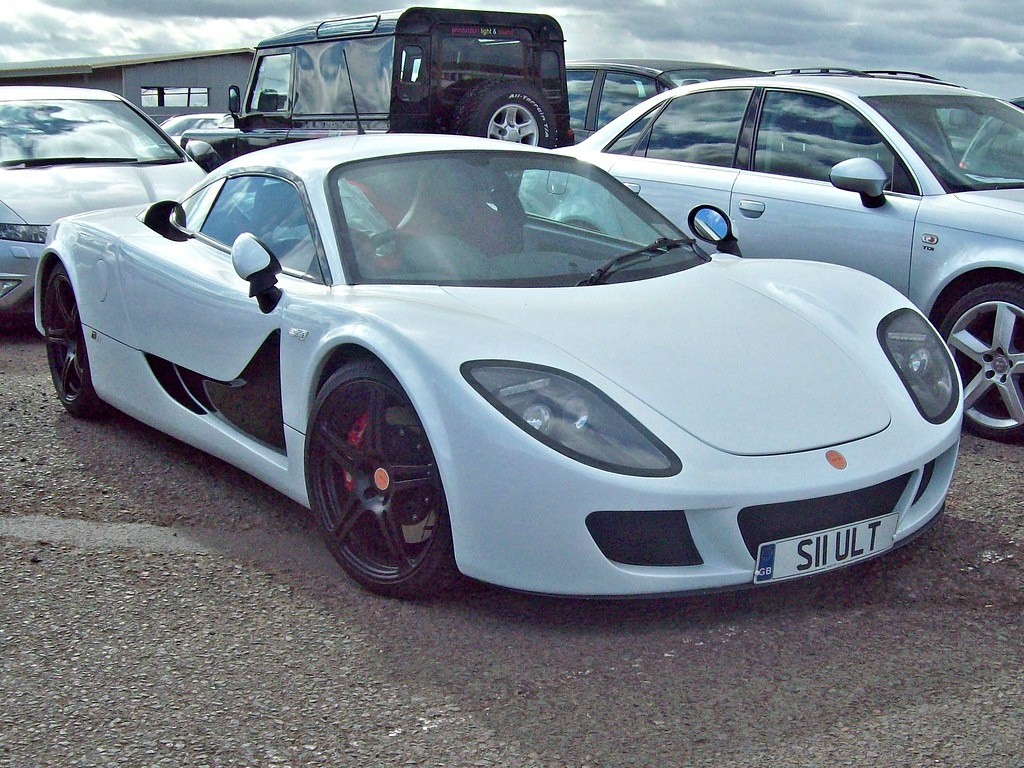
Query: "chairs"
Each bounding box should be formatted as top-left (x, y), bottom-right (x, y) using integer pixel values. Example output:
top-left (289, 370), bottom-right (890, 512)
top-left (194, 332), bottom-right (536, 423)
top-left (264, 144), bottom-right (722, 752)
top-left (396, 158), bottom-right (519, 256)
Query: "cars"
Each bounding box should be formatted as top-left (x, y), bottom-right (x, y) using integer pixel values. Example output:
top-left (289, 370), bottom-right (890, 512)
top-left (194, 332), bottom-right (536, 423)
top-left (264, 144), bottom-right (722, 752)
top-left (141, 111), bottom-right (241, 172)
top-left (1, 84), bottom-right (230, 314)
top-left (565, 60), bottom-right (779, 145)
top-left (513, 66), bottom-right (1024, 438)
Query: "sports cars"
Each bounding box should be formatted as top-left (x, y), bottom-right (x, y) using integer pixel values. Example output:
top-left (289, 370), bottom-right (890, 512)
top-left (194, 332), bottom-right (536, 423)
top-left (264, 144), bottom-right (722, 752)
top-left (32, 132), bottom-right (963, 606)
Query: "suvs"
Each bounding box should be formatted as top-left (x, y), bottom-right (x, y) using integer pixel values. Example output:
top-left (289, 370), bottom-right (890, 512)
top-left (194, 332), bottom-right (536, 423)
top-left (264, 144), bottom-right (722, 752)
top-left (225, 6), bottom-right (574, 194)
